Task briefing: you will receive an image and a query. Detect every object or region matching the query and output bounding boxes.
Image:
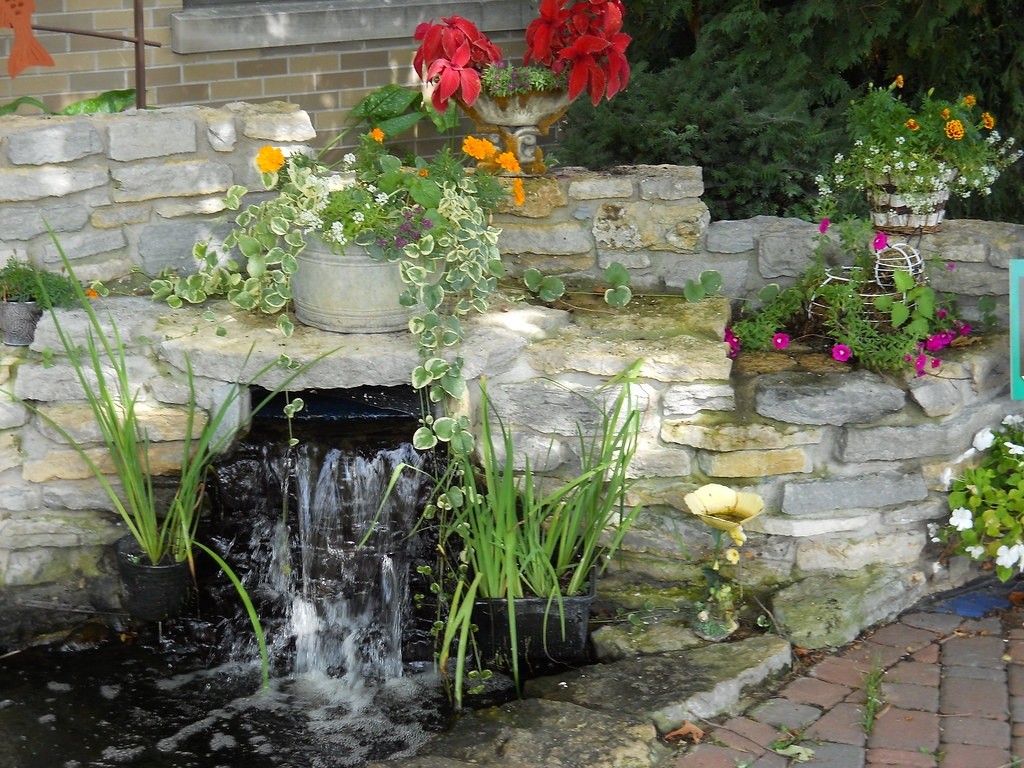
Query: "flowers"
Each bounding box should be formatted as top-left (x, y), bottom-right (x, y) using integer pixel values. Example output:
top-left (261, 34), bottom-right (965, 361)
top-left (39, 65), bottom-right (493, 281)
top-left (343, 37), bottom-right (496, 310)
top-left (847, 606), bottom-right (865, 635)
top-left (412, 0), bottom-right (631, 115)
top-left (256, 125), bottom-right (527, 253)
top-left (716, 217), bottom-right (969, 376)
top-left (928, 414), bottom-right (1024, 584)
top-left (683, 483), bottom-right (765, 621)
top-left (814, 74), bottom-right (1024, 199)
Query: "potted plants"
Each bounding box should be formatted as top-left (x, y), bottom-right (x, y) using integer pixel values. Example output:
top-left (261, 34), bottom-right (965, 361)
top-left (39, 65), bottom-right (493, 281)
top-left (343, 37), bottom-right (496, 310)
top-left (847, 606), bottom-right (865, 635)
top-left (437, 358), bottom-right (651, 707)
top-left (1, 215), bottom-right (344, 693)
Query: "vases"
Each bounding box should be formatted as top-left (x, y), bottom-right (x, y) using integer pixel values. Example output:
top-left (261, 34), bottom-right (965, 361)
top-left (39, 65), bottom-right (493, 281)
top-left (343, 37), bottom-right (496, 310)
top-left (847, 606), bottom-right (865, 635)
top-left (861, 160), bottom-right (961, 234)
top-left (432, 76), bottom-right (587, 176)
top-left (280, 226), bottom-right (436, 333)
